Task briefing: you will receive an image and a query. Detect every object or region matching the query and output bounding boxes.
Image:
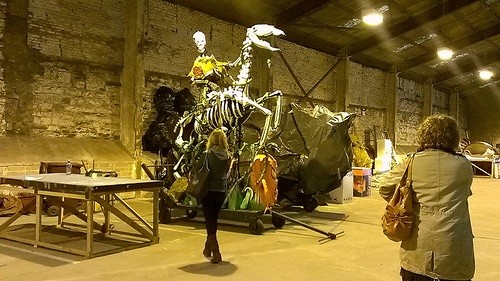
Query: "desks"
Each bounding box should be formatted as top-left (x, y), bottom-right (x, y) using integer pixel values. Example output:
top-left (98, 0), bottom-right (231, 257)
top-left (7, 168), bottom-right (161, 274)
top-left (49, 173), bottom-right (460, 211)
top-left (0, 173), bottom-right (165, 259)
top-left (469, 157), bottom-right (495, 179)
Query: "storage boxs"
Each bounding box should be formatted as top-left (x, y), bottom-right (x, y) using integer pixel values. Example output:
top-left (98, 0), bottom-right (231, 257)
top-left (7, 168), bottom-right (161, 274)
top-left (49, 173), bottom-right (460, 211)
top-left (327, 171), bottom-right (353, 204)
top-left (352, 167), bottom-right (372, 198)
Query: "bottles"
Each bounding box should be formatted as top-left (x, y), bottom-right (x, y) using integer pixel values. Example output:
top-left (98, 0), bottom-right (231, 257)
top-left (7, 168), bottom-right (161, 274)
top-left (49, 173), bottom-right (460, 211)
top-left (66, 159), bottom-right (72, 175)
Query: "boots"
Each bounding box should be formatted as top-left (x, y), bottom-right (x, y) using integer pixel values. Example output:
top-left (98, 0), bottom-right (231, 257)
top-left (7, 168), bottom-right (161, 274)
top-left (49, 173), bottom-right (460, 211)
top-left (203, 234), bottom-right (221, 263)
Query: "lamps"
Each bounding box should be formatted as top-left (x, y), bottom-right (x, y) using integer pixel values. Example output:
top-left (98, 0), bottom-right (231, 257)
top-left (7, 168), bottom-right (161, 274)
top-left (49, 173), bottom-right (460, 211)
top-left (361, 9), bottom-right (385, 25)
top-left (437, 47), bottom-right (453, 60)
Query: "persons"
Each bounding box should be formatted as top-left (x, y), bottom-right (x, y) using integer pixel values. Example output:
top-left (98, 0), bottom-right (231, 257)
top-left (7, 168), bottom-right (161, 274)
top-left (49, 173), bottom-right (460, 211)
top-left (196, 129), bottom-right (231, 263)
top-left (379, 116), bottom-right (475, 281)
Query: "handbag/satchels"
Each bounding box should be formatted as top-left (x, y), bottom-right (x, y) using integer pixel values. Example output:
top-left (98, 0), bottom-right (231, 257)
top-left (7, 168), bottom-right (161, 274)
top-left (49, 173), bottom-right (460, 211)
top-left (186, 153), bottom-right (210, 197)
top-left (381, 153), bottom-right (417, 242)
